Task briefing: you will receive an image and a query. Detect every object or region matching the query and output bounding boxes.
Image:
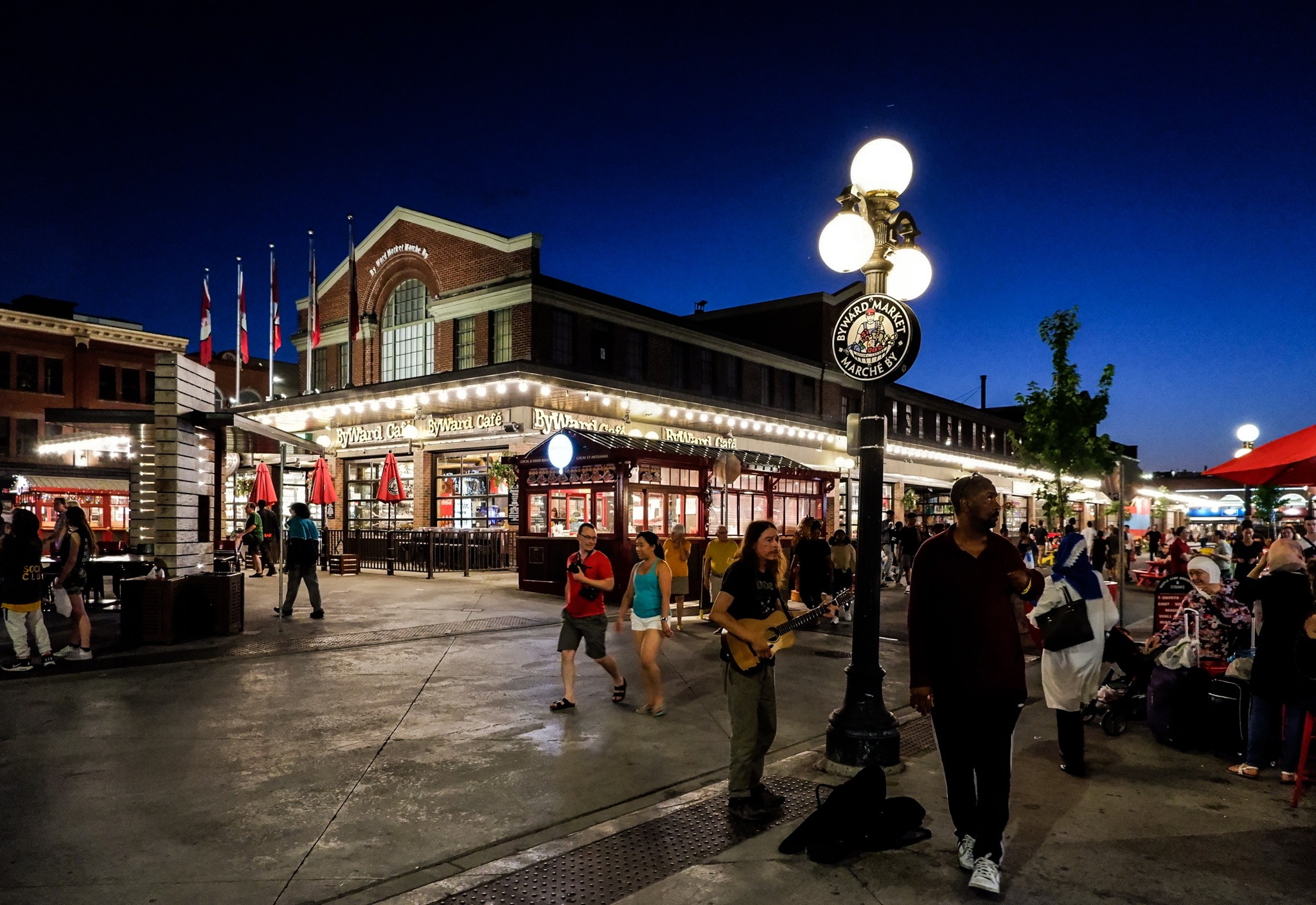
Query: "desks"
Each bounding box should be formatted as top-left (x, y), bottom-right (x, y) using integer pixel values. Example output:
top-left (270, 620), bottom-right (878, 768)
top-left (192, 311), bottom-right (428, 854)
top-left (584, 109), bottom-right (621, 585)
top-left (39, 552), bottom-right (159, 605)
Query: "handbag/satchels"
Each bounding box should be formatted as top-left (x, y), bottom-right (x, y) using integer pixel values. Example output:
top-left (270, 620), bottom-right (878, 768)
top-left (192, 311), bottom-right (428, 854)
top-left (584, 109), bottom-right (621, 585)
top-left (777, 760), bottom-right (933, 866)
top-left (1034, 585), bottom-right (1095, 652)
top-left (53, 578), bottom-right (72, 617)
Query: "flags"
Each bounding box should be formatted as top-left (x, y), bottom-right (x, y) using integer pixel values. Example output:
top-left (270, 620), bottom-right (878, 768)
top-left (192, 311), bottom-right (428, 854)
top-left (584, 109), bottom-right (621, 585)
top-left (200, 223), bottom-right (358, 365)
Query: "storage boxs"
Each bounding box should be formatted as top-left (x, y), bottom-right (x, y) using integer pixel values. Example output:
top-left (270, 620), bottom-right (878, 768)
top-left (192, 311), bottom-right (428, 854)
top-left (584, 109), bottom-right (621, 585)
top-left (1146, 608), bottom-right (1214, 753)
top-left (1206, 604), bottom-right (1258, 758)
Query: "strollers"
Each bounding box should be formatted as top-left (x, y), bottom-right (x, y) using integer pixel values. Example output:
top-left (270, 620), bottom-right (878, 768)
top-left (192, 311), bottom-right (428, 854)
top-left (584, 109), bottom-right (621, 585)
top-left (1081, 626), bottom-right (1163, 737)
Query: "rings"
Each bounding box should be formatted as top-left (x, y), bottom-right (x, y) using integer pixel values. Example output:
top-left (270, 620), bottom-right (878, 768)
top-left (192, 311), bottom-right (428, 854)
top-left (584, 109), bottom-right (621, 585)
top-left (915, 705), bottom-right (919, 708)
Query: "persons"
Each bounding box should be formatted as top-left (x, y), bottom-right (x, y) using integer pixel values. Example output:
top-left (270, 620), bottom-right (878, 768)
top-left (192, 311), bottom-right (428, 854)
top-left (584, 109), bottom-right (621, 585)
top-left (549, 522), bottom-right (627, 712)
top-left (551, 507), bottom-right (560, 527)
top-left (904, 473), bottom-right (1045, 899)
top-left (232, 501), bottom-right (264, 578)
top-left (663, 516), bottom-right (1316, 633)
top-left (615, 532), bottom-right (673, 717)
top-left (257, 500), bottom-right (280, 576)
top-left (279, 502), bottom-right (295, 574)
top-left (273, 503), bottom-right (325, 619)
top-left (40, 497), bottom-right (69, 556)
top-left (67, 501), bottom-right (83, 508)
top-left (52, 507), bottom-right (100, 661)
top-left (0, 508), bottom-right (56, 671)
top-left (1025, 534), bottom-right (1122, 779)
top-left (1225, 539), bottom-right (1315, 785)
top-left (1143, 557), bottom-right (1253, 748)
top-left (709, 521), bottom-right (839, 828)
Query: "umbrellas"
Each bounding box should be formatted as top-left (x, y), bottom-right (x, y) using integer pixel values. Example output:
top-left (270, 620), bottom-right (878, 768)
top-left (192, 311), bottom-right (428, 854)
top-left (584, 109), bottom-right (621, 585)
top-left (375, 449), bottom-right (407, 530)
top-left (309, 454), bottom-right (338, 530)
top-left (246, 458), bottom-right (278, 510)
top-left (1201, 425), bottom-right (1315, 486)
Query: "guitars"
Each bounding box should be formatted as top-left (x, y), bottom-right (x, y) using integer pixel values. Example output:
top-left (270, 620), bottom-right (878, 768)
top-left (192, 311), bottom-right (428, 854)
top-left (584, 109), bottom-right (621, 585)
top-left (720, 587), bottom-right (855, 676)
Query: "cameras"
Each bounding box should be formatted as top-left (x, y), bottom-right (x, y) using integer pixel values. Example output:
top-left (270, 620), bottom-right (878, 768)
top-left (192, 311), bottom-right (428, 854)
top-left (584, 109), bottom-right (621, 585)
top-left (568, 562), bottom-right (586, 573)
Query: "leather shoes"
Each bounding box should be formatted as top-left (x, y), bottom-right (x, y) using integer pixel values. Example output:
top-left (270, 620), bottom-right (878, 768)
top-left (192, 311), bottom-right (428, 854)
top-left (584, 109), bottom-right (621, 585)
top-left (274, 606), bottom-right (293, 616)
top-left (310, 612), bottom-right (323, 619)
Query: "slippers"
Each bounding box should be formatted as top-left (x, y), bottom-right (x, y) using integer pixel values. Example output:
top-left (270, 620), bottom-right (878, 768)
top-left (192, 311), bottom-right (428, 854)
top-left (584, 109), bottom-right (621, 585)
top-left (635, 703), bottom-right (653, 713)
top-left (651, 704), bottom-right (664, 717)
top-left (612, 676), bottom-right (627, 703)
top-left (550, 697), bottom-right (575, 710)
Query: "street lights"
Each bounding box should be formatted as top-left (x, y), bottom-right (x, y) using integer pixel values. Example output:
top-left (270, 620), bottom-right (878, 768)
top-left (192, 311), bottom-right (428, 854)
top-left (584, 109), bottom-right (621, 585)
top-left (1234, 424), bottom-right (1259, 521)
top-left (818, 139), bottom-right (933, 768)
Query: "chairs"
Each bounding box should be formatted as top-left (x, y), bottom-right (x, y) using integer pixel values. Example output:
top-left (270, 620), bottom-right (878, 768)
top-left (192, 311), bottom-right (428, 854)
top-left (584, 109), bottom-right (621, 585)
top-left (90, 541), bottom-right (127, 604)
top-left (135, 543), bottom-right (155, 554)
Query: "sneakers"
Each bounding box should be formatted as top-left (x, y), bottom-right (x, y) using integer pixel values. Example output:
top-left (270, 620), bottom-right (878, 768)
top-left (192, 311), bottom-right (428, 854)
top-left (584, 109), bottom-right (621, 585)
top-left (278, 568), bottom-right (289, 573)
top-left (266, 568), bottom-right (277, 576)
top-left (727, 797), bottom-right (768, 820)
top-left (0, 656), bottom-right (34, 672)
top-left (967, 852), bottom-right (1002, 894)
top-left (53, 644), bottom-right (80, 657)
top-left (64, 647), bottom-right (93, 661)
top-left (41, 653), bottom-right (56, 666)
top-left (957, 834), bottom-right (976, 870)
top-left (752, 781), bottom-right (786, 809)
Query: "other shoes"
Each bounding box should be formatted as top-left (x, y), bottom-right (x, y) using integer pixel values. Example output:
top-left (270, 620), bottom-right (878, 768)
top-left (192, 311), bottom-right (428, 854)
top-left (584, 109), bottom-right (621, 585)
top-left (1061, 762), bottom-right (1089, 778)
top-left (660, 570), bottom-right (911, 633)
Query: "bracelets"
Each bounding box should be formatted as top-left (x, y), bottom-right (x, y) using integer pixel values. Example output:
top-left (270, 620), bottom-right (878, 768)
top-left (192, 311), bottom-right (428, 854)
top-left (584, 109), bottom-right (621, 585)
top-left (1256, 563), bottom-right (1263, 571)
top-left (661, 617), bottom-right (668, 621)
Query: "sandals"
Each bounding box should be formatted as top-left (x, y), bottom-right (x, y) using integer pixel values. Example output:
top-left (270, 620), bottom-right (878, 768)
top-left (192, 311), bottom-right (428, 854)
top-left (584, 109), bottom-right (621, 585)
top-left (1279, 770), bottom-right (1312, 784)
top-left (249, 572), bottom-right (263, 577)
top-left (1225, 763), bottom-right (1259, 778)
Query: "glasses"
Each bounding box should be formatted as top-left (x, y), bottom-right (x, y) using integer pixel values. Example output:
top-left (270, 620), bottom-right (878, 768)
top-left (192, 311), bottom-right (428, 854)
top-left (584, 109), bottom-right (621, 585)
top-left (717, 531), bottom-right (729, 534)
top-left (958, 471), bottom-right (984, 503)
top-left (578, 532), bottom-right (599, 540)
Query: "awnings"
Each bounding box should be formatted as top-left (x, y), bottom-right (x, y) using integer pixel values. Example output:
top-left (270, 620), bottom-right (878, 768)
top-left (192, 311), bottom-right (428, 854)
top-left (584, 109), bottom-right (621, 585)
top-left (43, 408), bottom-right (155, 443)
top-left (194, 411), bottom-right (323, 454)
